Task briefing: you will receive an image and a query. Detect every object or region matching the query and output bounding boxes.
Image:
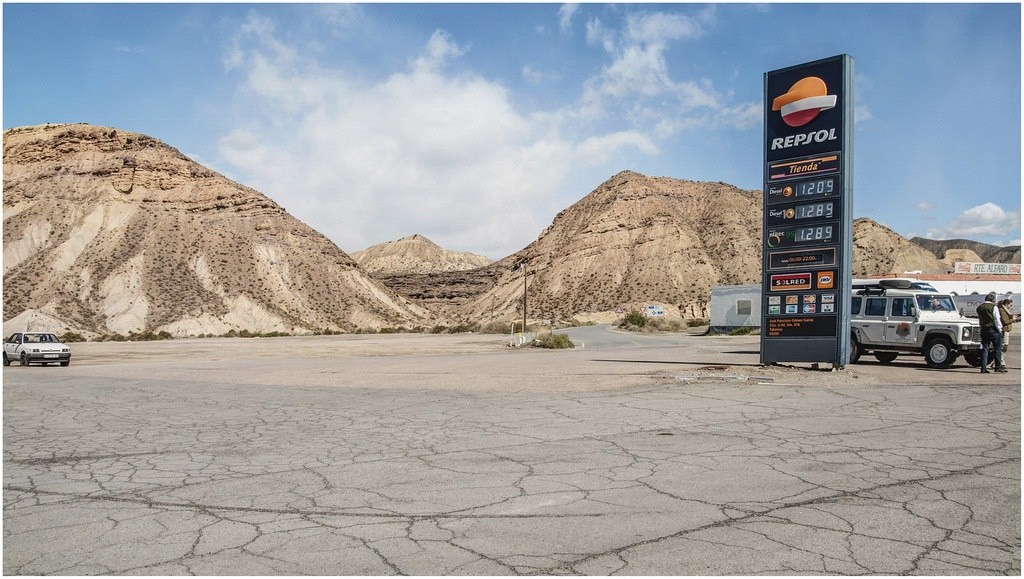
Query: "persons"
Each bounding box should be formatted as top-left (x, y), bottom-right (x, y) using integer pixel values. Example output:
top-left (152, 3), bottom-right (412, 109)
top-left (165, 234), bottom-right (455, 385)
top-left (975, 295), bottom-right (1006, 373)
top-left (992, 299), bottom-right (1021, 368)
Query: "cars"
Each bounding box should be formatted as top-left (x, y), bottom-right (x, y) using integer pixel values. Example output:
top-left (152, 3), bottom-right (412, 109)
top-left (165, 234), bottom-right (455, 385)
top-left (3, 332), bottom-right (72, 368)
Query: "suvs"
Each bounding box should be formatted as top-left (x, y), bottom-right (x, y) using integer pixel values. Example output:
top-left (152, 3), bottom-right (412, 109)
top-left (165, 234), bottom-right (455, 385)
top-left (846, 277), bottom-right (1007, 370)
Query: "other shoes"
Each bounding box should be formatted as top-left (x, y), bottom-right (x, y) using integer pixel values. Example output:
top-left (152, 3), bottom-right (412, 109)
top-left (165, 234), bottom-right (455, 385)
top-left (981, 369), bottom-right (990, 373)
top-left (995, 366), bottom-right (1009, 373)
